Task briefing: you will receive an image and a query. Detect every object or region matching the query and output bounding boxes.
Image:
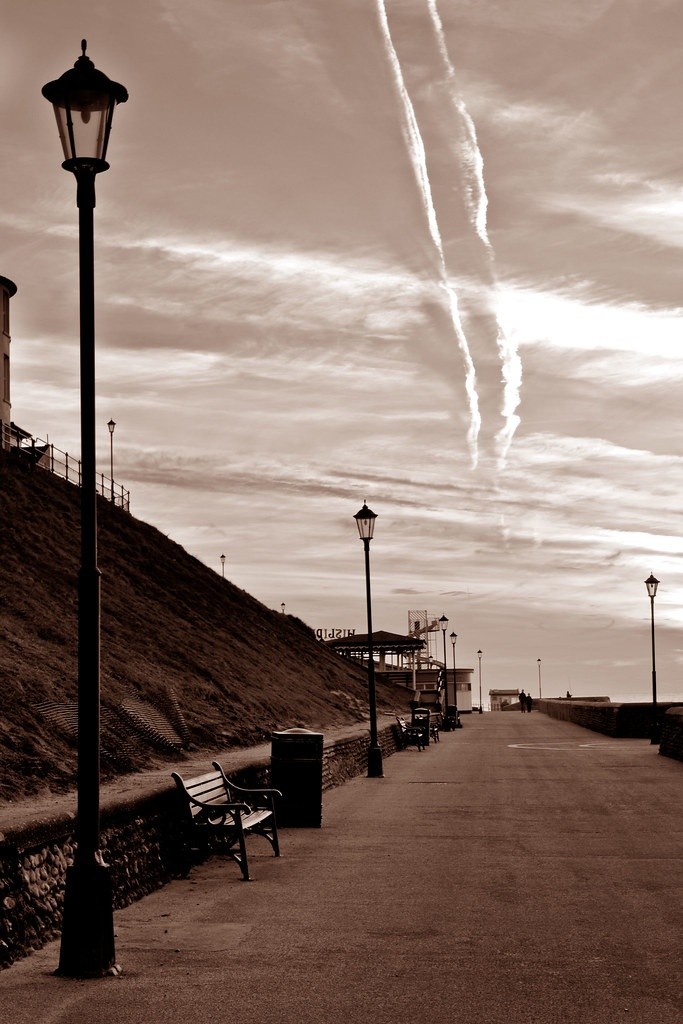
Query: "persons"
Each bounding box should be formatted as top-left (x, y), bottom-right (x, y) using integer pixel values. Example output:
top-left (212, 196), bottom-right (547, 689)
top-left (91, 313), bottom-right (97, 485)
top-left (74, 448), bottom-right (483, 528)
top-left (519, 689), bottom-right (526, 713)
top-left (526, 693), bottom-right (533, 713)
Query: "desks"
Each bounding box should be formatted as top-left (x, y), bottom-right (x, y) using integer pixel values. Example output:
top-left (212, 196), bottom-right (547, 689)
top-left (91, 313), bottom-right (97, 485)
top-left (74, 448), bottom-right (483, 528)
top-left (412, 718), bottom-right (440, 743)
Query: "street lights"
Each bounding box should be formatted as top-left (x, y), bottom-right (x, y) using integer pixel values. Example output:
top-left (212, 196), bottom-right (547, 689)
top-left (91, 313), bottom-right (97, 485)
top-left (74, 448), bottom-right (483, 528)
top-left (477, 650), bottom-right (483, 714)
top-left (439, 613), bottom-right (449, 731)
top-left (537, 659), bottom-right (541, 699)
top-left (353, 499), bottom-right (383, 778)
top-left (450, 632), bottom-right (458, 727)
top-left (644, 571), bottom-right (662, 745)
top-left (42, 39), bottom-right (129, 982)
top-left (220, 554), bottom-right (226, 578)
top-left (107, 418), bottom-right (116, 503)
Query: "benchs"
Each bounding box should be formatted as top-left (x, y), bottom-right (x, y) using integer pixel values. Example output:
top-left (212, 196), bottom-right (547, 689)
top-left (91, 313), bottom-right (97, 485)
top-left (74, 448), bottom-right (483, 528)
top-left (171, 762), bottom-right (282, 880)
top-left (396, 716), bottom-right (425, 752)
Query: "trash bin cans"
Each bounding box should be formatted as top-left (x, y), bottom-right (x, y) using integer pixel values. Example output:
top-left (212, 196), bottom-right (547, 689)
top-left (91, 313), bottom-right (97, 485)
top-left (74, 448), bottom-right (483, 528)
top-left (271, 728), bottom-right (324, 830)
top-left (411, 707), bottom-right (430, 747)
top-left (447, 705), bottom-right (457, 731)
